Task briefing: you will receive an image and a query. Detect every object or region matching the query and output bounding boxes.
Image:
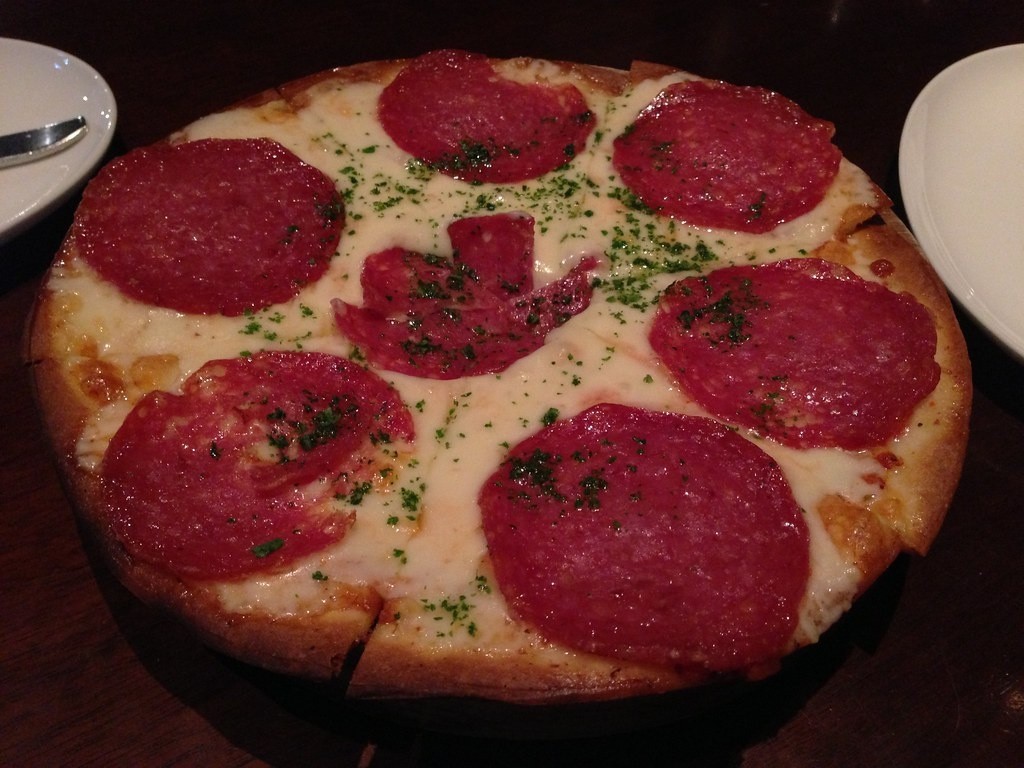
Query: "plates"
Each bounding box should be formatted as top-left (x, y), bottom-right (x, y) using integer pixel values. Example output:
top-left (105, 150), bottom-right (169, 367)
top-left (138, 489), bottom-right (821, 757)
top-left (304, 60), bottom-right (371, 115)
top-left (896, 42), bottom-right (1023, 362)
top-left (0, 35), bottom-right (119, 245)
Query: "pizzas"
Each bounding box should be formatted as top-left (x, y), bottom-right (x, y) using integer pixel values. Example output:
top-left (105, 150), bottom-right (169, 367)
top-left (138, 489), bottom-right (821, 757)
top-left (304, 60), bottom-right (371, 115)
top-left (15, 48), bottom-right (974, 707)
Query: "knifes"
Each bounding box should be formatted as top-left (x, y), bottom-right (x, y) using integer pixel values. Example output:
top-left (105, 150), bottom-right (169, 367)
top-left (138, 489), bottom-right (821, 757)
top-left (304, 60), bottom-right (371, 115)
top-left (0, 115), bottom-right (87, 171)
